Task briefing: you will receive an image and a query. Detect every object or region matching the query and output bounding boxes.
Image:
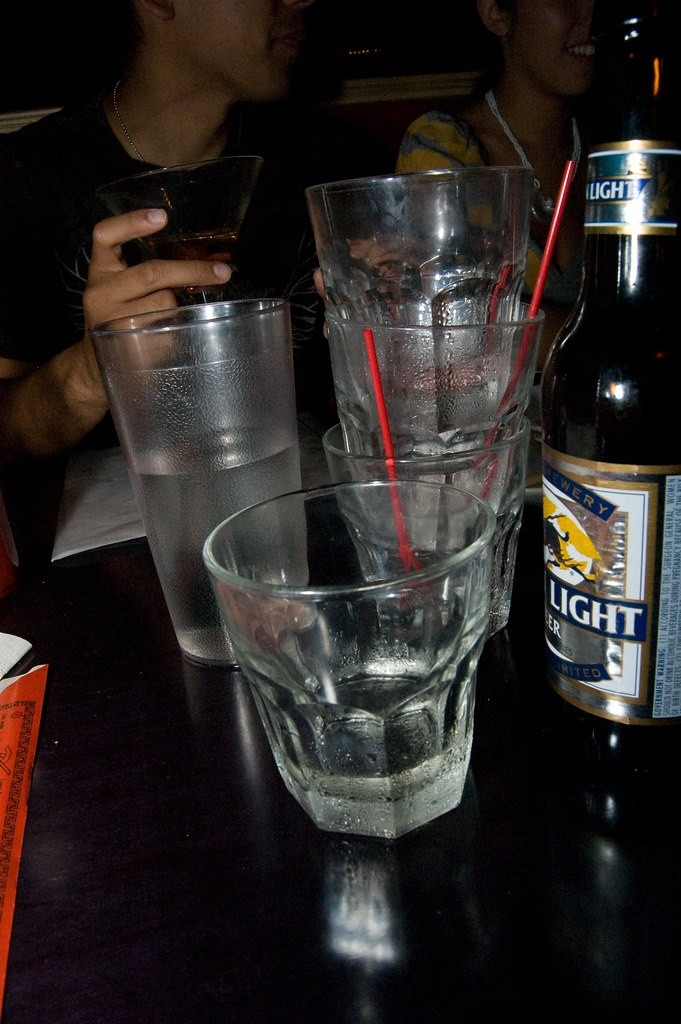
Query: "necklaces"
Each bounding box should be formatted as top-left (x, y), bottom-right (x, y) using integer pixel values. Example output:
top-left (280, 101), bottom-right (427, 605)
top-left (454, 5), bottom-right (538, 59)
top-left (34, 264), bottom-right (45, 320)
top-left (114, 80), bottom-right (175, 210)
top-left (486, 89), bottom-right (582, 226)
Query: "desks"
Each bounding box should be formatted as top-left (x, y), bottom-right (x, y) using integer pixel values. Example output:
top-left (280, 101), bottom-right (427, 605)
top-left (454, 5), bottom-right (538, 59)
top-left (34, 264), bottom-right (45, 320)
top-left (0, 385), bottom-right (681, 1024)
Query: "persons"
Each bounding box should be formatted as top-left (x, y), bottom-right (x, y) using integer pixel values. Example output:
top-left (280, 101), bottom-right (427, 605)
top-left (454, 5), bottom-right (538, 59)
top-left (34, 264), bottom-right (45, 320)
top-left (395, 0), bottom-right (617, 375)
top-left (1, 0), bottom-right (418, 454)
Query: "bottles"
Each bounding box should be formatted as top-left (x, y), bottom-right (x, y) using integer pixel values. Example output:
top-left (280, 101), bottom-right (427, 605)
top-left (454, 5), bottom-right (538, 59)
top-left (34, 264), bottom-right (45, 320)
top-left (540, 0), bottom-right (681, 837)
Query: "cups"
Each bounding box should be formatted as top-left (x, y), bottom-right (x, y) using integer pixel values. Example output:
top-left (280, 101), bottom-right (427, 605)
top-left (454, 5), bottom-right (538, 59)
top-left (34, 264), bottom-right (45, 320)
top-left (323, 303), bottom-right (545, 637)
top-left (206, 484), bottom-right (495, 838)
top-left (92, 302), bottom-right (310, 668)
top-left (323, 414), bottom-right (534, 637)
top-left (305, 166), bottom-right (535, 303)
top-left (94, 155), bottom-right (264, 303)
